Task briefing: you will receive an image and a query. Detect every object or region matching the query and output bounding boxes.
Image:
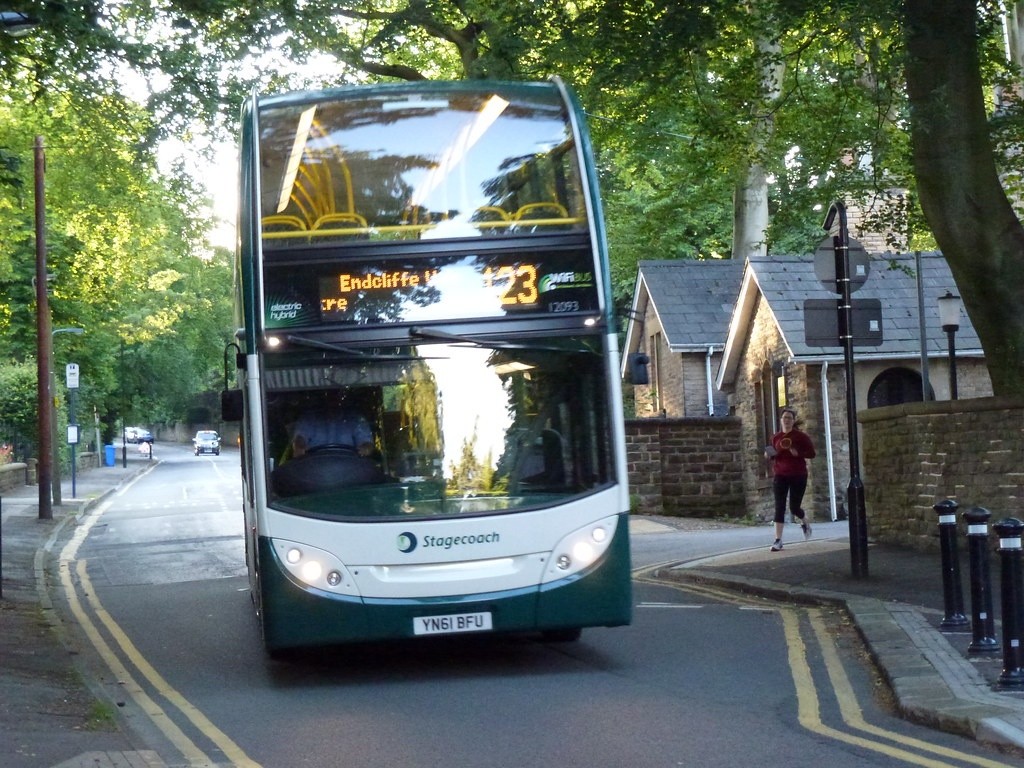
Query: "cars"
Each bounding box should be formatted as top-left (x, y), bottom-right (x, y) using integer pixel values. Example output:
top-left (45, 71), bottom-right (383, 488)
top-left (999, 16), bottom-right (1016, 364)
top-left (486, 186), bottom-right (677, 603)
top-left (192, 428), bottom-right (225, 458)
top-left (127, 427), bottom-right (151, 441)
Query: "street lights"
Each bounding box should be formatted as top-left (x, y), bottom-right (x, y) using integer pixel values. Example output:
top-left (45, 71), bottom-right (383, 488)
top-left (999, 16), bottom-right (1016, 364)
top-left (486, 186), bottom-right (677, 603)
top-left (47, 328), bottom-right (84, 507)
top-left (937, 290), bottom-right (961, 400)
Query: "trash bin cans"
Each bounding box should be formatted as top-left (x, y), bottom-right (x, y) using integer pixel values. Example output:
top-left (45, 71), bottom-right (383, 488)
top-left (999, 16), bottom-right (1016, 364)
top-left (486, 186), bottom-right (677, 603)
top-left (105, 445), bottom-right (116, 467)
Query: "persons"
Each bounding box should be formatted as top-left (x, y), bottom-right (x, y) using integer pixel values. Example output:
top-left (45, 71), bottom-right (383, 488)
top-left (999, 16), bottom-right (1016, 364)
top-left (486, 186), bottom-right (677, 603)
top-left (763, 409), bottom-right (816, 551)
top-left (290, 385), bottom-right (376, 464)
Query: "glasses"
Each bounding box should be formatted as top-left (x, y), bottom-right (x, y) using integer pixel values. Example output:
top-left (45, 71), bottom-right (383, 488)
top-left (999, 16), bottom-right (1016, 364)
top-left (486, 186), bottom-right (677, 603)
top-left (781, 415), bottom-right (793, 420)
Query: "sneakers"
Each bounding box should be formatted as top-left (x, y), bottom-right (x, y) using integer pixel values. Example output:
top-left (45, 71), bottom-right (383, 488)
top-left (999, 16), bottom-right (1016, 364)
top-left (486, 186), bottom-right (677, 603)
top-left (770, 539), bottom-right (783, 551)
top-left (800, 516), bottom-right (812, 539)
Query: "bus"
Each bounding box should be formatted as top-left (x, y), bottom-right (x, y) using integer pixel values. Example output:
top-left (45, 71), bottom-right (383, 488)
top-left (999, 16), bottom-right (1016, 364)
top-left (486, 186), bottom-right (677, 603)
top-left (221, 74), bottom-right (647, 659)
